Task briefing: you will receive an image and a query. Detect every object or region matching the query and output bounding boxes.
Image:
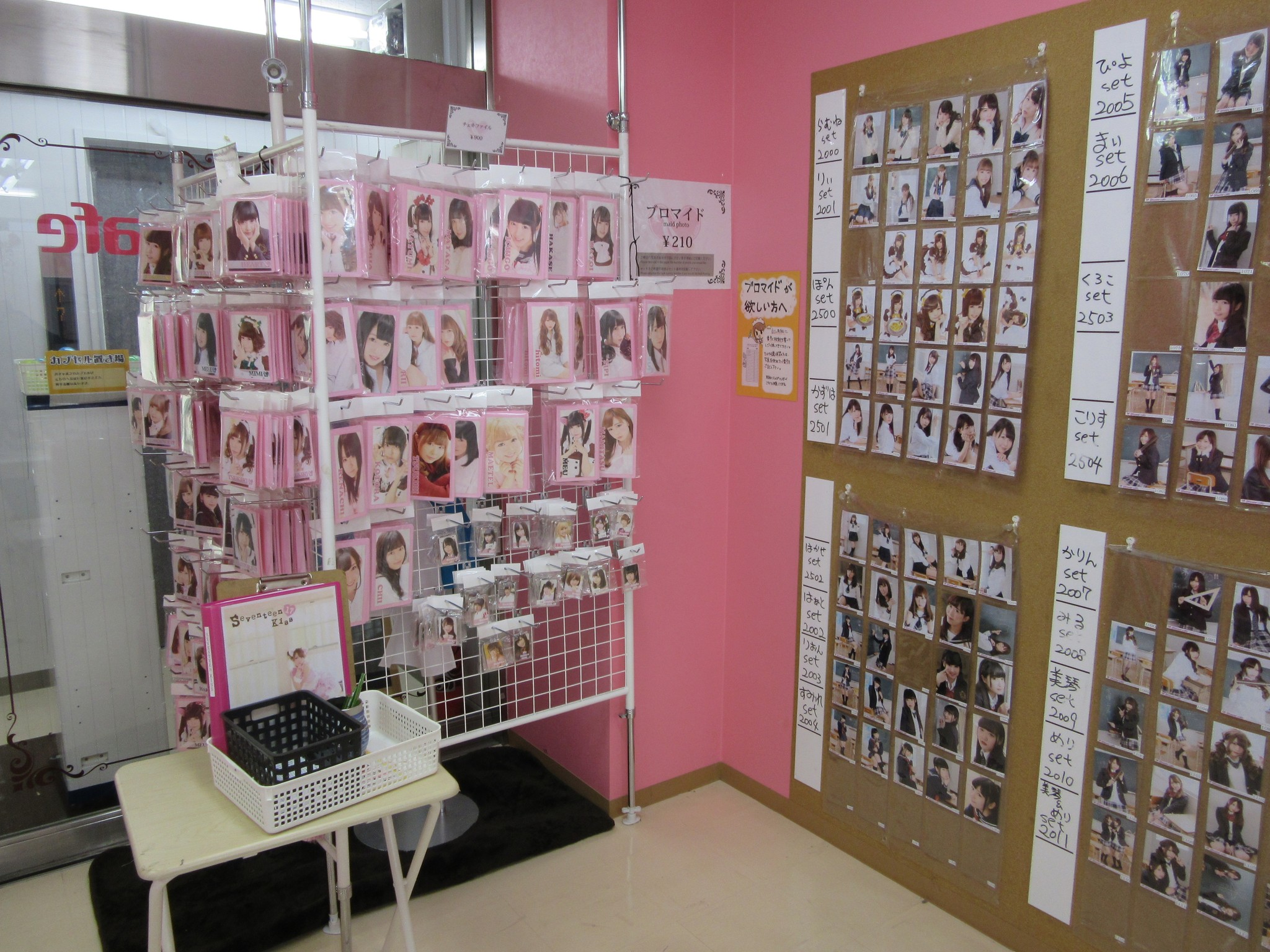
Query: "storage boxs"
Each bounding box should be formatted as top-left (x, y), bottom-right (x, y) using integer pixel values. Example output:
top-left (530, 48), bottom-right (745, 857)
top-left (205, 689), bottom-right (442, 834)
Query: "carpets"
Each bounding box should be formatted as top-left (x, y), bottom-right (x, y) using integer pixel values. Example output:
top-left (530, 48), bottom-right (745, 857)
top-left (91, 746), bottom-right (614, 952)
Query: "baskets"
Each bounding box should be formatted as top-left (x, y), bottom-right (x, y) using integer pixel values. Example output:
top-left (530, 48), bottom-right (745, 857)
top-left (221, 688), bottom-right (363, 787)
top-left (205, 690), bottom-right (440, 835)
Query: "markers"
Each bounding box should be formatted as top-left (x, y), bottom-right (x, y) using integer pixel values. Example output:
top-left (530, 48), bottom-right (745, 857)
top-left (342, 694), bottom-right (351, 710)
top-left (351, 673), bottom-right (366, 708)
top-left (354, 700), bottom-right (360, 707)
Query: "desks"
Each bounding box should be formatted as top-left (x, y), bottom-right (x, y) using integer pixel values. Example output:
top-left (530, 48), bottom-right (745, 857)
top-left (114, 743), bottom-right (459, 952)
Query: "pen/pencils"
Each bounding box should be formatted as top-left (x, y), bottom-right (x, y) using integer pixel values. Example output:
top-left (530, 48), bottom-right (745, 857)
top-left (346, 686), bottom-right (357, 709)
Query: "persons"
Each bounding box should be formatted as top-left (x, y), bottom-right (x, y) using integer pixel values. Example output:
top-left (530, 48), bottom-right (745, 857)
top-left (828, 28), bottom-right (1270, 945)
top-left (642, 305), bottom-right (668, 375)
top-left (124, 179), bottom-right (638, 753)
